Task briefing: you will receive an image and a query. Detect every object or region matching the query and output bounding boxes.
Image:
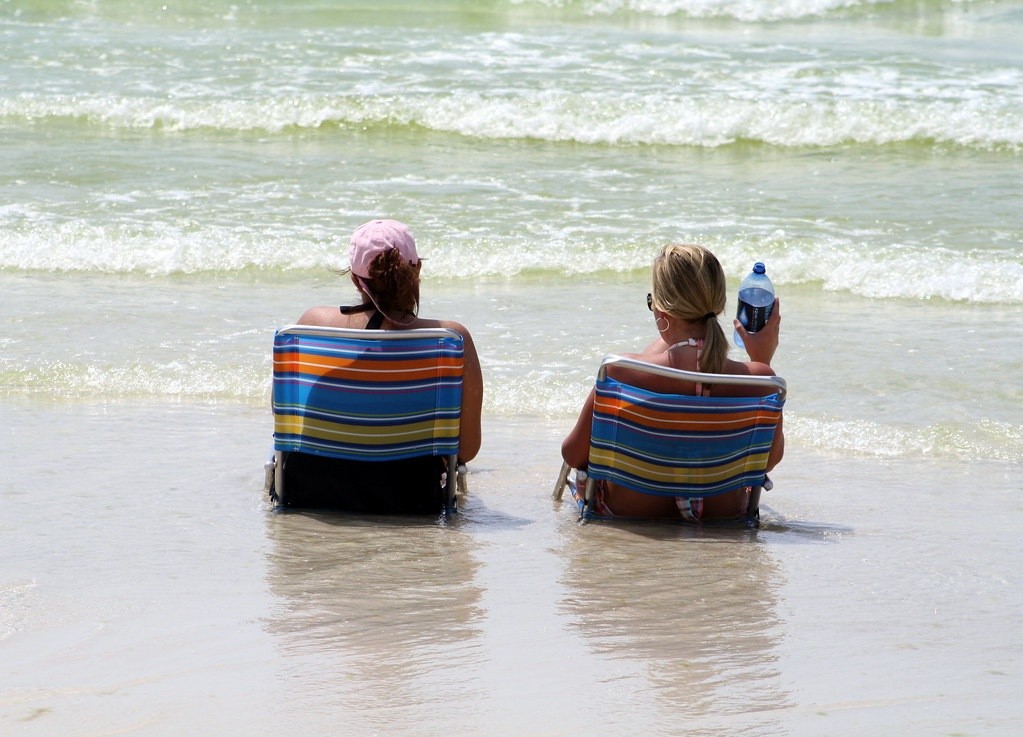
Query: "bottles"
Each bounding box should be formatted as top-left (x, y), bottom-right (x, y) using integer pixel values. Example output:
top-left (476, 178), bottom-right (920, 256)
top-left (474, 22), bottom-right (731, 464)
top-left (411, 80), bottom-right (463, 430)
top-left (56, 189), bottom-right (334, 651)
top-left (734, 262), bottom-right (774, 350)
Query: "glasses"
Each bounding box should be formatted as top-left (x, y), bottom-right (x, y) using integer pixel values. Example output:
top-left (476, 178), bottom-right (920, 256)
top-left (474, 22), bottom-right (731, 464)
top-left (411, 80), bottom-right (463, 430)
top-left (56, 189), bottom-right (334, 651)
top-left (647, 293), bottom-right (654, 311)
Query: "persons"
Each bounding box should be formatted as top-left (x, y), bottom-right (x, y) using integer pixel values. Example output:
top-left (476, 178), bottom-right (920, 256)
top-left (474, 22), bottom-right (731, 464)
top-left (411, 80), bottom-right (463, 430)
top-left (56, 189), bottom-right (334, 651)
top-left (270, 218), bottom-right (484, 462)
top-left (559, 242), bottom-right (785, 473)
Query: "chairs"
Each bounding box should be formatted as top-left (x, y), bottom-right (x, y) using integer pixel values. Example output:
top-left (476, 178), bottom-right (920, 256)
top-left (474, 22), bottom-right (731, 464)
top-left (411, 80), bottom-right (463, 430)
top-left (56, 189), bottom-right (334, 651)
top-left (264, 325), bottom-right (464, 524)
top-left (552, 354), bottom-right (787, 532)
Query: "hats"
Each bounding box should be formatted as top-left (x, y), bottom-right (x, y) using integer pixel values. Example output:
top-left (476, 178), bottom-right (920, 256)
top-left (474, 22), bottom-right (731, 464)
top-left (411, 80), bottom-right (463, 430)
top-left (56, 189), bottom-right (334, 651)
top-left (348, 219), bottom-right (417, 278)
